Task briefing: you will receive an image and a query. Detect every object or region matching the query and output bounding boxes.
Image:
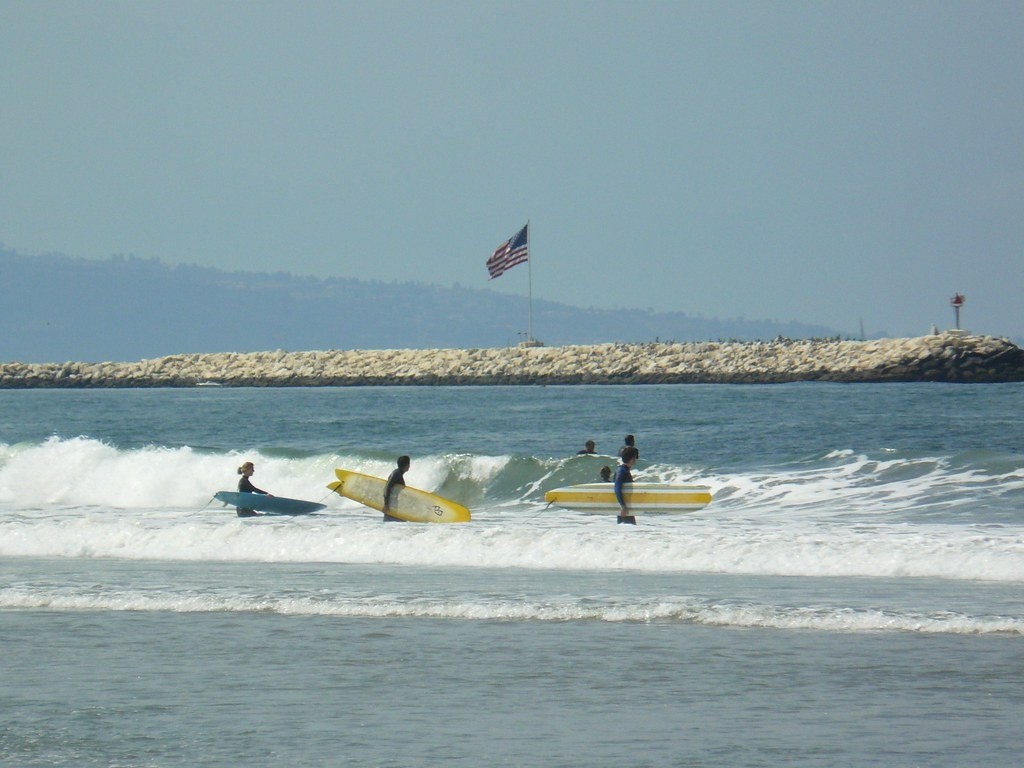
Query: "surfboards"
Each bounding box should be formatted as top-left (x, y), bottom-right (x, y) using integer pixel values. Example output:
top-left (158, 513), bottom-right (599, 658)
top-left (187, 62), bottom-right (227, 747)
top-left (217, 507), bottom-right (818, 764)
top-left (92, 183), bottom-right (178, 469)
top-left (543, 482), bottom-right (713, 517)
top-left (325, 468), bottom-right (472, 524)
top-left (213, 490), bottom-right (328, 516)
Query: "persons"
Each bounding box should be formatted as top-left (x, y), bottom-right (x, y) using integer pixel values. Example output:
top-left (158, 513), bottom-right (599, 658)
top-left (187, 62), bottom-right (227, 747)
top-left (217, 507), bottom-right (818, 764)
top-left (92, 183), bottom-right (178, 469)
top-left (614, 447), bottom-right (636, 525)
top-left (576, 441), bottom-right (598, 454)
top-left (599, 465), bottom-right (612, 482)
top-left (618, 435), bottom-right (638, 458)
top-left (382, 456), bottom-right (410, 521)
top-left (237, 462), bottom-right (273, 517)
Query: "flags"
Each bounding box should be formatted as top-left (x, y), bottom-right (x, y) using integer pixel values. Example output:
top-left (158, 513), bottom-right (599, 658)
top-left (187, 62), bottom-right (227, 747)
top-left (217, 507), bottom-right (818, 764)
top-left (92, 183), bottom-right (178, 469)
top-left (487, 224), bottom-right (529, 279)
